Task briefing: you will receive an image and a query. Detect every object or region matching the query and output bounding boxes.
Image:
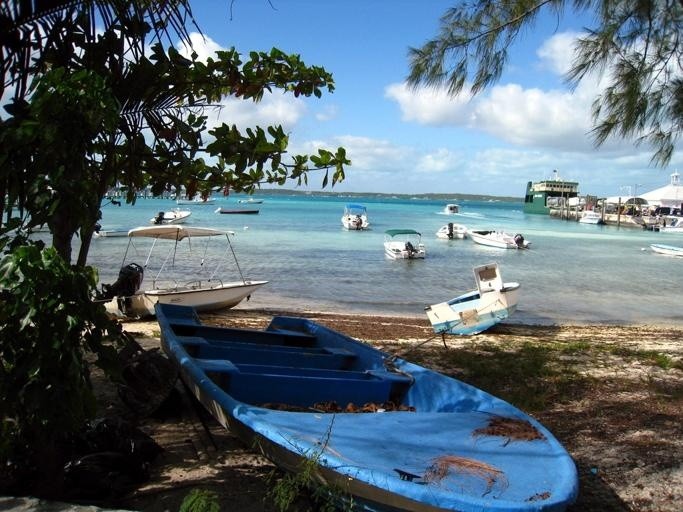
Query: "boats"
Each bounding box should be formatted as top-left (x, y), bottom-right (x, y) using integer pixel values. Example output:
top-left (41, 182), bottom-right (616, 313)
top-left (19, 220), bottom-right (51, 234)
top-left (153, 301), bottom-right (581, 512)
top-left (434, 222), bottom-right (533, 250)
top-left (424, 262), bottom-right (521, 336)
top-left (149, 190), bottom-right (265, 227)
top-left (341, 204), bottom-right (369, 231)
top-left (383, 228), bottom-right (426, 260)
top-left (444, 203), bottom-right (460, 214)
top-left (522, 168), bottom-right (580, 215)
top-left (91, 223), bottom-right (270, 321)
top-left (649, 243), bottom-right (683, 256)
